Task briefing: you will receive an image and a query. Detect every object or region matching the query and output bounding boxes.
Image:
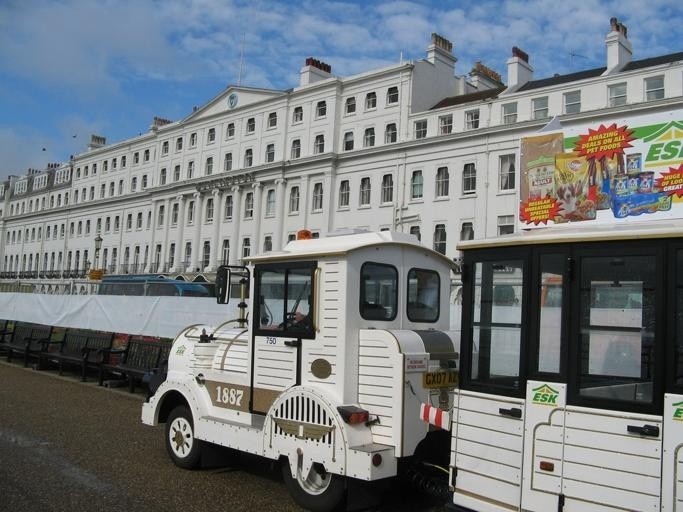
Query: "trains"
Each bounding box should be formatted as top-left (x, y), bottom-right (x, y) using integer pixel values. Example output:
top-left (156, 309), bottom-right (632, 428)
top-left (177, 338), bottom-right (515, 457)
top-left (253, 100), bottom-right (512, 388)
top-left (141, 219), bottom-right (683, 512)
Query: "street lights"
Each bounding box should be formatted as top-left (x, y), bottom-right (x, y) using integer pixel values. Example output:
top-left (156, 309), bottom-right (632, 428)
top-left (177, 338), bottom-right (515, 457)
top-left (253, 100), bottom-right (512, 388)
top-left (94, 233), bottom-right (103, 270)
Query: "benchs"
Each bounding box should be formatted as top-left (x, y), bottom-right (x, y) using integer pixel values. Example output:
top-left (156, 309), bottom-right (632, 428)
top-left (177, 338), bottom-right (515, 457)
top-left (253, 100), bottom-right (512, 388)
top-left (0, 320), bottom-right (171, 402)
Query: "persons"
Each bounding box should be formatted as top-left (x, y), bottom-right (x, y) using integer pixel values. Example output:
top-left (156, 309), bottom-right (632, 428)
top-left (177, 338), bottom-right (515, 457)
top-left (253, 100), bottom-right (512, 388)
top-left (295, 312), bottom-right (303, 322)
